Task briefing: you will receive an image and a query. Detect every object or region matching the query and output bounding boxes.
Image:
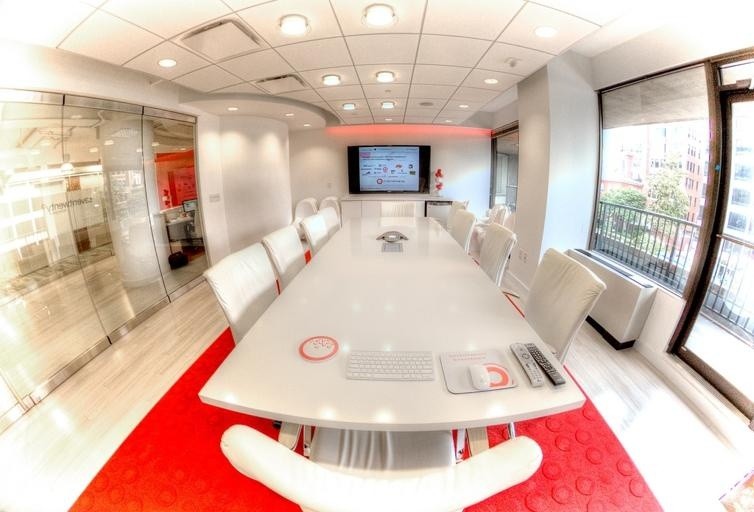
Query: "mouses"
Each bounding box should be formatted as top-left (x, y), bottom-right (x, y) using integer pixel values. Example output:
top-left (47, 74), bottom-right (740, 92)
top-left (468, 363), bottom-right (490, 390)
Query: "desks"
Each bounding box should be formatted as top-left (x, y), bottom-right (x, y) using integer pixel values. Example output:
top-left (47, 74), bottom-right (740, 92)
top-left (194, 217), bottom-right (587, 432)
top-left (166, 216), bottom-right (194, 242)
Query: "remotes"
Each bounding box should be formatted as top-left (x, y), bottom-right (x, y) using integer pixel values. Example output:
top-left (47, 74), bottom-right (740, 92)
top-left (508, 343), bottom-right (544, 388)
top-left (524, 343), bottom-right (565, 387)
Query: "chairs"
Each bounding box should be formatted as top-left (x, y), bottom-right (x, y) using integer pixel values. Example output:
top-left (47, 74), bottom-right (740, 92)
top-left (220, 422), bottom-right (543, 512)
top-left (446, 199), bottom-right (607, 367)
top-left (192, 208), bottom-right (203, 250)
top-left (381, 201), bottom-right (416, 219)
top-left (202, 196), bottom-right (341, 349)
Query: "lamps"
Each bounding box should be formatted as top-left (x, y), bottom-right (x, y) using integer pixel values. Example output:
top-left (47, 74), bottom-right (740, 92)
top-left (280, 2), bottom-right (396, 110)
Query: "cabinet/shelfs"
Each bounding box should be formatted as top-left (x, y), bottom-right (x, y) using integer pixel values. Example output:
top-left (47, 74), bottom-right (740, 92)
top-left (339, 195), bottom-right (455, 218)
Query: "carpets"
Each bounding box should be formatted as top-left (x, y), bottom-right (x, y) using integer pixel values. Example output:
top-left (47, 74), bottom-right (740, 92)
top-left (66, 250), bottom-right (665, 512)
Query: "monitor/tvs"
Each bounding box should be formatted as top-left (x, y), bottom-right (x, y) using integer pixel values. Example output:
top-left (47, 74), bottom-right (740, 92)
top-left (182, 197), bottom-right (197, 214)
top-left (348, 146), bottom-right (430, 194)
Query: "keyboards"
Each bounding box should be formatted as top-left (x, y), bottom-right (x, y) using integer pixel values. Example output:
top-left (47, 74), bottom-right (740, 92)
top-left (346, 351), bottom-right (436, 382)
